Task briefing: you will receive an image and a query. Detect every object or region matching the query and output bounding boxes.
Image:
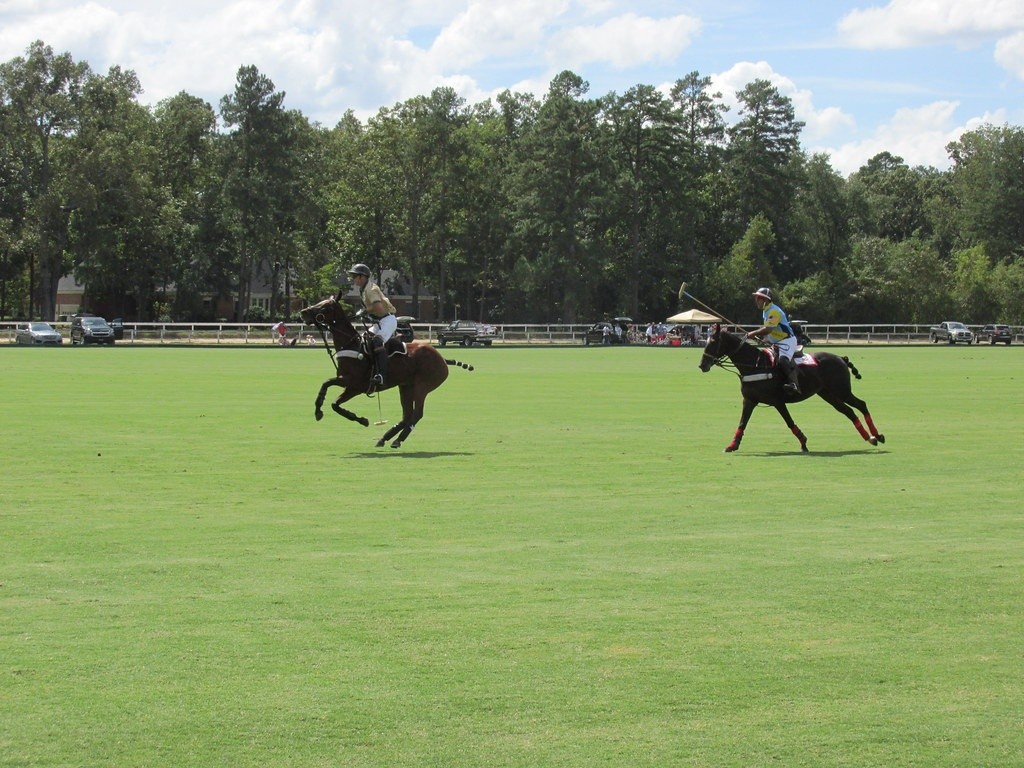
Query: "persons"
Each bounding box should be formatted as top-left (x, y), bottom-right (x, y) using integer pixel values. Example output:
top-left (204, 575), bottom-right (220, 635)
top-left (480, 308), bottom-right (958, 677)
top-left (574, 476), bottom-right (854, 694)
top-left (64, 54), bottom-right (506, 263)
top-left (603, 322), bottom-right (727, 345)
top-left (277, 321), bottom-right (286, 343)
top-left (747, 287), bottom-right (797, 406)
top-left (348, 263), bottom-right (398, 383)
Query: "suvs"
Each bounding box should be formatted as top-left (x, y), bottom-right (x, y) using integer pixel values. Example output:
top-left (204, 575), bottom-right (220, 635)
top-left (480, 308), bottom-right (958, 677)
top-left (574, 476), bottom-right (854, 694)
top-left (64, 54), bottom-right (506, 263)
top-left (974, 323), bottom-right (1012, 345)
top-left (71, 313), bottom-right (124, 347)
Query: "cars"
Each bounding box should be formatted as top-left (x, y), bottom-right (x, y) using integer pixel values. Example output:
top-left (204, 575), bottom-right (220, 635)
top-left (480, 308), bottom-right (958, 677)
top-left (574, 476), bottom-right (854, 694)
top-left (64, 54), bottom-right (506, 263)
top-left (585, 316), bottom-right (633, 345)
top-left (15, 322), bottom-right (62, 347)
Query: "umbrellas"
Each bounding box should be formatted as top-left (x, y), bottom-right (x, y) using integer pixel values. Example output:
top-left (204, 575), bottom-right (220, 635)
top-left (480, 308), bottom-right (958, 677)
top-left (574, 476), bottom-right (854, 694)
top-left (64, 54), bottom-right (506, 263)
top-left (666, 309), bottom-right (722, 344)
top-left (615, 317), bottom-right (633, 324)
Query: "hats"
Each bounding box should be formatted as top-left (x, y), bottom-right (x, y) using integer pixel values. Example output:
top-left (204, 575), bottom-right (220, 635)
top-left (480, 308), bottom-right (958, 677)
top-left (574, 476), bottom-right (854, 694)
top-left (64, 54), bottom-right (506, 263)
top-left (752, 288), bottom-right (772, 300)
top-left (659, 322), bottom-right (662, 324)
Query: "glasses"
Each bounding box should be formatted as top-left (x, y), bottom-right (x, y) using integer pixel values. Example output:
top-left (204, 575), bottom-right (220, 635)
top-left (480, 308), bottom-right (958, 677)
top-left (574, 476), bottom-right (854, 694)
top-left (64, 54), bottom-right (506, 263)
top-left (353, 274), bottom-right (357, 278)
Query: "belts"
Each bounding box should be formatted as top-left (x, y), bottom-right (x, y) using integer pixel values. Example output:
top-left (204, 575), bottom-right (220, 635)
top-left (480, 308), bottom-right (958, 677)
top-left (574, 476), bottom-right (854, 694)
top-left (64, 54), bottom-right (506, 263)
top-left (783, 334), bottom-right (793, 339)
top-left (379, 313), bottom-right (393, 321)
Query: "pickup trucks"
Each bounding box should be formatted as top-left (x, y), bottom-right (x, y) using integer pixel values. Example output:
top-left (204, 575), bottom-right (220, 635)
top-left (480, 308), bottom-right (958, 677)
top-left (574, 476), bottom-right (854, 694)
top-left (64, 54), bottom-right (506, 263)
top-left (929, 321), bottom-right (974, 345)
top-left (437, 320), bottom-right (498, 347)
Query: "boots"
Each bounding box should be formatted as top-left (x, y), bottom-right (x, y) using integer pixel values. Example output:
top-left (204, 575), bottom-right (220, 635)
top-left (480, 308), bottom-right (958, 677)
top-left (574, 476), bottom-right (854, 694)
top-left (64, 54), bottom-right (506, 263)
top-left (779, 356), bottom-right (801, 394)
top-left (370, 337), bottom-right (388, 388)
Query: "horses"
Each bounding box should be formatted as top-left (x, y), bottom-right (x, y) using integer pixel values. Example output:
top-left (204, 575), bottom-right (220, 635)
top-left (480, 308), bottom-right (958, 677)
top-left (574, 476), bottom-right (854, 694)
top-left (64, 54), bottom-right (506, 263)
top-left (299, 289), bottom-right (474, 448)
top-left (698, 321), bottom-right (885, 451)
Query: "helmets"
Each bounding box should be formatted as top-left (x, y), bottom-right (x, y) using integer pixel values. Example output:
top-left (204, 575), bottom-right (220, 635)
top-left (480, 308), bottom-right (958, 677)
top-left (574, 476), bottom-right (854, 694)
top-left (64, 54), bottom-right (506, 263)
top-left (348, 264), bottom-right (370, 278)
